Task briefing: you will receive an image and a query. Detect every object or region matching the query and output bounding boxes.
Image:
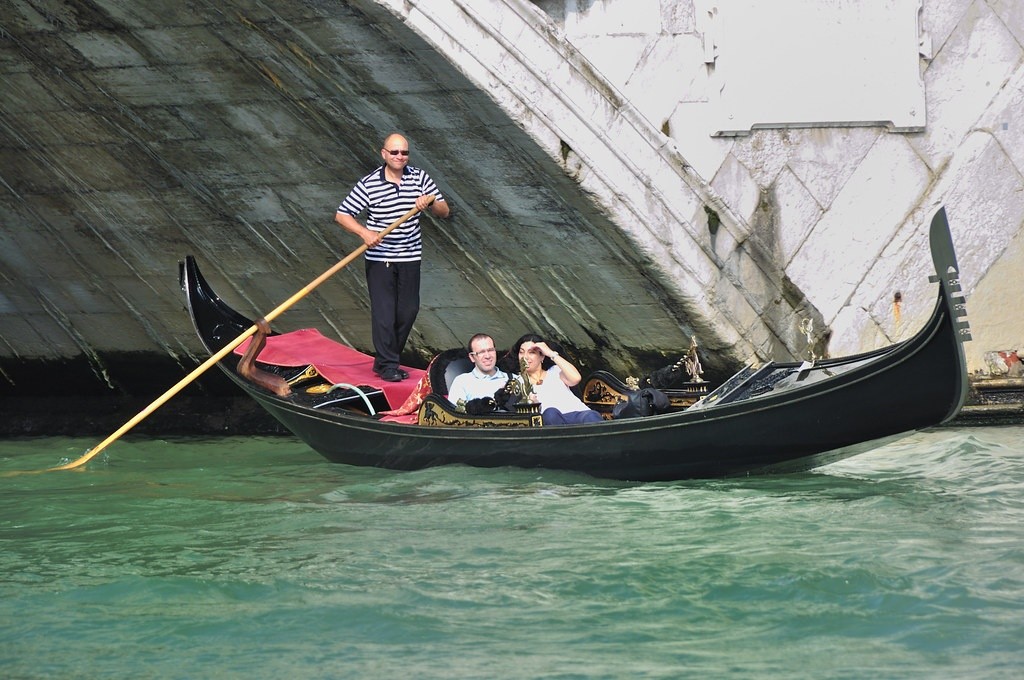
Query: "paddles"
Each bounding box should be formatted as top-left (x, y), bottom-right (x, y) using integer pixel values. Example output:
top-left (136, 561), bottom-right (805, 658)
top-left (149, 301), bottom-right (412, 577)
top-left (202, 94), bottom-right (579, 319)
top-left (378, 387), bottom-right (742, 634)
top-left (49, 192), bottom-right (437, 471)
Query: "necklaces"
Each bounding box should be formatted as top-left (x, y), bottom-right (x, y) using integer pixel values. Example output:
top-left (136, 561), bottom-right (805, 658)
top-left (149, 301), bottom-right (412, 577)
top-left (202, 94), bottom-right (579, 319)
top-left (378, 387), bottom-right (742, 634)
top-left (527, 369), bottom-right (543, 385)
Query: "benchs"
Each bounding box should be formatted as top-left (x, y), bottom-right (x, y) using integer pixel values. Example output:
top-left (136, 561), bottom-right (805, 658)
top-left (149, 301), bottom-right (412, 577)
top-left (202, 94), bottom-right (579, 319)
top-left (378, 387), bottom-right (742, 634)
top-left (417, 340), bottom-right (710, 428)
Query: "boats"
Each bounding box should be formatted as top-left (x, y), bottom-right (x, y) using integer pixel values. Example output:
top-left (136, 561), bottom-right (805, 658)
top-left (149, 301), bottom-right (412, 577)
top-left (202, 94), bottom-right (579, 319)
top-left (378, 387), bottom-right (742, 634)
top-left (178, 206), bottom-right (973, 482)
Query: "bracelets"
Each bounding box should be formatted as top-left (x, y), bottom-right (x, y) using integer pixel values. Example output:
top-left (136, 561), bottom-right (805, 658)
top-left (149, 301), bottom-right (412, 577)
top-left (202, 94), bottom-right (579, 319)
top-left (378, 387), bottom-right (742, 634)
top-left (550, 351), bottom-right (558, 360)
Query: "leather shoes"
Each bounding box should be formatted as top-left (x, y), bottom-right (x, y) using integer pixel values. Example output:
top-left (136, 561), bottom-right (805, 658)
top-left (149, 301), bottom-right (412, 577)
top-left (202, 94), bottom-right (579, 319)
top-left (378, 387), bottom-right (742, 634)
top-left (397, 370), bottom-right (408, 379)
top-left (377, 369), bottom-right (401, 381)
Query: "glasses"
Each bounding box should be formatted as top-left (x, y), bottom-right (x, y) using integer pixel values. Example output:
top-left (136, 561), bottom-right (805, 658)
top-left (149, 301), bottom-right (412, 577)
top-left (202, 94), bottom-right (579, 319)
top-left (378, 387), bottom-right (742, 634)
top-left (384, 148), bottom-right (410, 155)
top-left (470, 348), bottom-right (496, 356)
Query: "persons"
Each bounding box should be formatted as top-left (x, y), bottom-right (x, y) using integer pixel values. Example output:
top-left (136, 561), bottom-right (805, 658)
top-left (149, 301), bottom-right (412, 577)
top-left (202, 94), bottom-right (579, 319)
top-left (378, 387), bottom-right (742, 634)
top-left (334, 134), bottom-right (450, 382)
top-left (512, 334), bottom-right (604, 426)
top-left (446, 333), bottom-right (519, 413)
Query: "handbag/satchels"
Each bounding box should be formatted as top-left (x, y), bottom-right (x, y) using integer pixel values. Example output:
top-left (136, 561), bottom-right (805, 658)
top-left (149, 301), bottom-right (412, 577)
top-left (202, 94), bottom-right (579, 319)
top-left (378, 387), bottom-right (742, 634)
top-left (615, 387), bottom-right (671, 418)
